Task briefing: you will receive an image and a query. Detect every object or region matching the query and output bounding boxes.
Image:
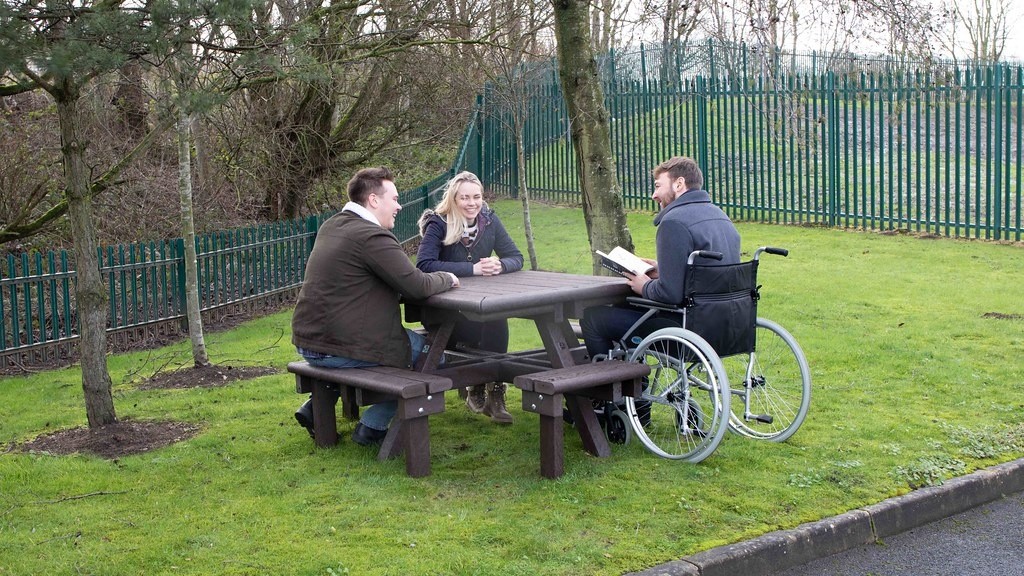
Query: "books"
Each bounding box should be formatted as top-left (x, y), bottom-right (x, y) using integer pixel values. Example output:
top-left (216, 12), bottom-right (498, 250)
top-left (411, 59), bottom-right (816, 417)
top-left (596, 246), bottom-right (655, 281)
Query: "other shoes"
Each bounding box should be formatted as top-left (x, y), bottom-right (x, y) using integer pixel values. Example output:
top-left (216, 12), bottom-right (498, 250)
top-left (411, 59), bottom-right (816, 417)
top-left (633, 402), bottom-right (652, 425)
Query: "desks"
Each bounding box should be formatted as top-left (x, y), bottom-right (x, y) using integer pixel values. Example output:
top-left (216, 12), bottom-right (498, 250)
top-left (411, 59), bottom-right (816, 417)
top-left (376, 270), bottom-right (633, 462)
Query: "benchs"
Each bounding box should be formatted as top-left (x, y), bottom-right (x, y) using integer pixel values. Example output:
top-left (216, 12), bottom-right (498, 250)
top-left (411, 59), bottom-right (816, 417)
top-left (516, 360), bottom-right (652, 483)
top-left (287, 359), bottom-right (453, 477)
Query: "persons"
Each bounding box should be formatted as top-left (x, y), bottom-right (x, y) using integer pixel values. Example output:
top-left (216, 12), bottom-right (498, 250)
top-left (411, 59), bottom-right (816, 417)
top-left (579, 157), bottom-right (741, 428)
top-left (292, 168), bottom-right (461, 447)
top-left (415, 171), bottom-right (524, 423)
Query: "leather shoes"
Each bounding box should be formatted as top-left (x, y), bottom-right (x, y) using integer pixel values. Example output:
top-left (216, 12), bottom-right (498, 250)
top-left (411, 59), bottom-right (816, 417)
top-left (351, 422), bottom-right (388, 445)
top-left (295, 397), bottom-right (341, 439)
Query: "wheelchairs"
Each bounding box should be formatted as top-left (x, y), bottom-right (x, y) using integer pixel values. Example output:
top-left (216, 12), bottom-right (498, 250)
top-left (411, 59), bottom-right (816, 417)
top-left (562, 246), bottom-right (812, 465)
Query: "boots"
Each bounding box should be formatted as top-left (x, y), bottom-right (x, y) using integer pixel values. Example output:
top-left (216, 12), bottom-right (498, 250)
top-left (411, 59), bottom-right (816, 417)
top-left (482, 383), bottom-right (513, 423)
top-left (466, 385), bottom-right (486, 414)
